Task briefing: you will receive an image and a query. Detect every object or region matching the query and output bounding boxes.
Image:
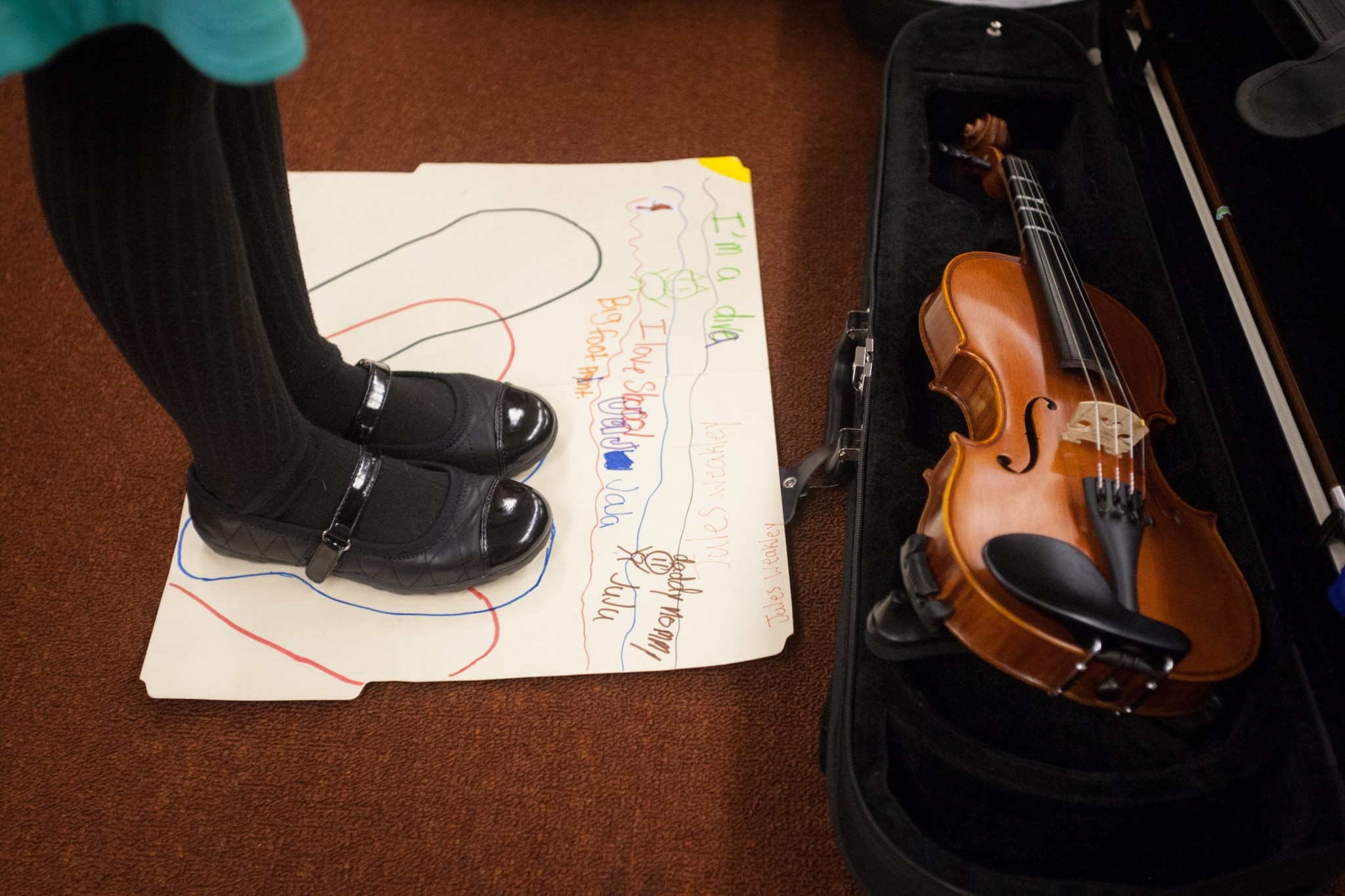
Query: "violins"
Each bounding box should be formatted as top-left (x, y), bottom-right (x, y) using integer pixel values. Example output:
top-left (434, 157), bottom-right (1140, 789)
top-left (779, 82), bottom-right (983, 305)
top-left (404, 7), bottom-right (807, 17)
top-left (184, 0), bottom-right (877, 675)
top-left (899, 111), bottom-right (1263, 721)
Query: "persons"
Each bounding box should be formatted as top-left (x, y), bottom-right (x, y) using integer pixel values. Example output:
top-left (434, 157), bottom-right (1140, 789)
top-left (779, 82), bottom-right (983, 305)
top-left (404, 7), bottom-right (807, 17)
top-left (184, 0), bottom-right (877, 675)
top-left (1, 0), bottom-right (559, 594)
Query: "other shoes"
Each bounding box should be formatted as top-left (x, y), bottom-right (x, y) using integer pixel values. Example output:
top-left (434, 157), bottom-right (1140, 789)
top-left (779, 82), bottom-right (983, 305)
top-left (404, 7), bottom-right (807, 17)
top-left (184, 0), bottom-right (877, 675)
top-left (185, 444), bottom-right (553, 597)
top-left (341, 357), bottom-right (558, 479)
